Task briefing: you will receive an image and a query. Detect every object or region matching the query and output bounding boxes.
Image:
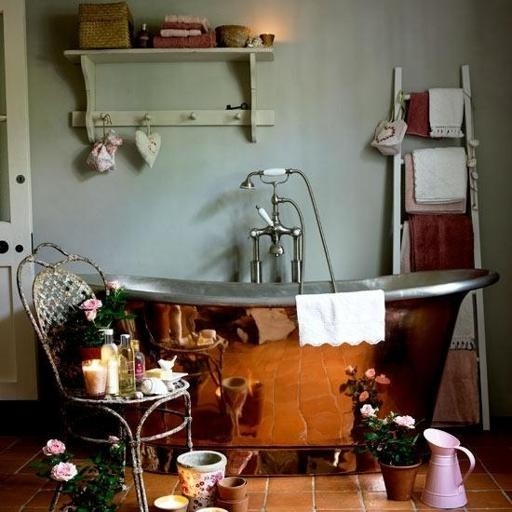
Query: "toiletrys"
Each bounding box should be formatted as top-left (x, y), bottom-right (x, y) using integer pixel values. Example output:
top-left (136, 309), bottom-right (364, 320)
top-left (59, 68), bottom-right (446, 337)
top-left (142, 377), bottom-right (170, 398)
top-left (98, 328), bottom-right (116, 398)
top-left (117, 332), bottom-right (136, 395)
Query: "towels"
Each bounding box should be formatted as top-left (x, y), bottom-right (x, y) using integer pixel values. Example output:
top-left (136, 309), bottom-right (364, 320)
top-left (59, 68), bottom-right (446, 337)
top-left (448, 289), bottom-right (474, 350)
top-left (247, 305), bottom-right (294, 346)
top-left (425, 87), bottom-right (465, 138)
top-left (432, 349), bottom-right (481, 426)
top-left (404, 153), bottom-right (466, 219)
top-left (405, 88), bottom-right (430, 138)
top-left (411, 211), bottom-right (475, 273)
top-left (399, 221), bottom-right (411, 274)
top-left (153, 13), bottom-right (213, 48)
top-left (412, 147), bottom-right (468, 203)
top-left (293, 288), bottom-right (387, 346)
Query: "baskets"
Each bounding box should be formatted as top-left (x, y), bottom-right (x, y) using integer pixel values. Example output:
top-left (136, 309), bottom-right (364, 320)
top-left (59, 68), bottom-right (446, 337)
top-left (215, 25), bottom-right (250, 47)
top-left (79, 16), bottom-right (130, 49)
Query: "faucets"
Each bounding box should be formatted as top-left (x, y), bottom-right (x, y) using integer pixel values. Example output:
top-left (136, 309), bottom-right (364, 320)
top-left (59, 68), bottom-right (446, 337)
top-left (246, 204), bottom-right (303, 285)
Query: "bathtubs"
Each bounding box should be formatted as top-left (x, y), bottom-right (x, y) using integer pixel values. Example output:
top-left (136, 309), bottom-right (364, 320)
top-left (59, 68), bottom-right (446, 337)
top-left (65, 267), bottom-right (503, 477)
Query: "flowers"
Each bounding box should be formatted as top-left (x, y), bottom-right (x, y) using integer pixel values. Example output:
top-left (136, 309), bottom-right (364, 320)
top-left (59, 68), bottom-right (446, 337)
top-left (29, 432), bottom-right (134, 510)
top-left (339, 363), bottom-right (389, 433)
top-left (360, 399), bottom-right (422, 463)
top-left (60, 276), bottom-right (135, 345)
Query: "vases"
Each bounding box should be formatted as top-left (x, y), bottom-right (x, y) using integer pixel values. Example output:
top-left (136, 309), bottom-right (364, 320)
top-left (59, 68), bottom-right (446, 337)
top-left (152, 446), bottom-right (253, 512)
top-left (353, 442), bottom-right (383, 472)
top-left (349, 396), bottom-right (384, 442)
top-left (380, 456), bottom-right (420, 500)
top-left (80, 341), bottom-right (103, 368)
top-left (212, 376), bottom-right (265, 438)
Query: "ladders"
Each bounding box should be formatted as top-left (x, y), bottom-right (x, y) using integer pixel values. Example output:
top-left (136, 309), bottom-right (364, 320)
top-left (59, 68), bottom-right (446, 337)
top-left (392, 65), bottom-right (490, 431)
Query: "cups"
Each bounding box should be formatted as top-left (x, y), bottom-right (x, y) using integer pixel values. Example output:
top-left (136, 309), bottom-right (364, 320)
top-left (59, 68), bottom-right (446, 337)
top-left (241, 380), bottom-right (266, 425)
top-left (81, 359), bottom-right (107, 398)
top-left (154, 495), bottom-right (188, 512)
top-left (219, 375), bottom-right (248, 413)
top-left (178, 449), bottom-right (226, 512)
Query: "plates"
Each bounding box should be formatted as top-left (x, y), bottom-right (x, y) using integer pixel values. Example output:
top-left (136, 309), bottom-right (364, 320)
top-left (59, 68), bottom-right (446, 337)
top-left (146, 367), bottom-right (190, 383)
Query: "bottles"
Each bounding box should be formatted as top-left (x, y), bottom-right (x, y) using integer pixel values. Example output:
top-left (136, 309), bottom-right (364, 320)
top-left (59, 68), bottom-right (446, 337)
top-left (101, 330), bottom-right (144, 394)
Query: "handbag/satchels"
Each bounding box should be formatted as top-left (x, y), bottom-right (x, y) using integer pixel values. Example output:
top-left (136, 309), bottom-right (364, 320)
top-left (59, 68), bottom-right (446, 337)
top-left (370, 121), bottom-right (408, 156)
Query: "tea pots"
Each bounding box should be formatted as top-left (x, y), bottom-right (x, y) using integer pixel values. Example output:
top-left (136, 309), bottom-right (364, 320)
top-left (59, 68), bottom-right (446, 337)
top-left (421, 429), bottom-right (474, 510)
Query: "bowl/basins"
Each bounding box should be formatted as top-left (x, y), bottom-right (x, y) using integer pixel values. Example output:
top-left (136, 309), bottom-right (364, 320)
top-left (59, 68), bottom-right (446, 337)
top-left (215, 497), bottom-right (250, 512)
top-left (217, 476), bottom-right (250, 498)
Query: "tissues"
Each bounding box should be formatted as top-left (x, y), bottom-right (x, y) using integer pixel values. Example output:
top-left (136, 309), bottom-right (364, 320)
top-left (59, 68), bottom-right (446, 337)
top-left (146, 355), bottom-right (178, 379)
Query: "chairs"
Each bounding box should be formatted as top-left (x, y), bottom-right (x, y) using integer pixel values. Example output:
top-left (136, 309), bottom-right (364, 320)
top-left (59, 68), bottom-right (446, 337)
top-left (137, 306), bottom-right (229, 452)
top-left (15, 241), bottom-right (194, 510)
